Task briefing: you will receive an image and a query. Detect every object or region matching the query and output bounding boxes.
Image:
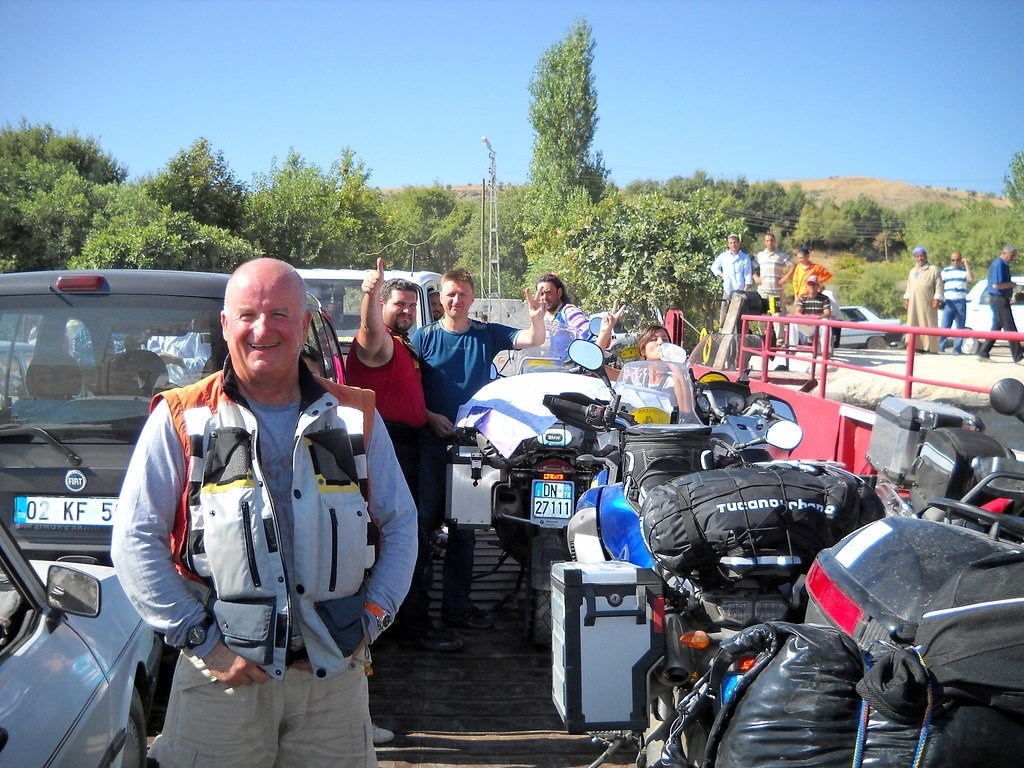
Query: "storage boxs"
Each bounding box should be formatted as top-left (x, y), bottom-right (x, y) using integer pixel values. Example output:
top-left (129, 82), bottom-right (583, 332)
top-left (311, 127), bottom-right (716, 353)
top-left (804, 517), bottom-right (1024, 660)
top-left (865, 394), bottom-right (1017, 515)
top-left (547, 561), bottom-right (664, 733)
top-left (622, 423), bottom-right (712, 505)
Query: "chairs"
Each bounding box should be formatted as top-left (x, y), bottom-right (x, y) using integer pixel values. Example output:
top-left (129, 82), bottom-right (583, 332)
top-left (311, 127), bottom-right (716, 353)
top-left (11, 347), bottom-right (169, 422)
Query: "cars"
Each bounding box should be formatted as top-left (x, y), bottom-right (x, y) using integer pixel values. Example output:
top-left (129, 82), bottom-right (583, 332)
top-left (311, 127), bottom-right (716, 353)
top-left (0, 513), bottom-right (165, 768)
top-left (938, 275), bottom-right (1024, 356)
top-left (295, 268), bottom-right (445, 364)
top-left (0, 267), bottom-right (343, 571)
top-left (836, 305), bottom-right (902, 351)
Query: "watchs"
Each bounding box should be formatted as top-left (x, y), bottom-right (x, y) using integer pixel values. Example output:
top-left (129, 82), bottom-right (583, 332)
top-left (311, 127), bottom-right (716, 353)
top-left (185, 616), bottom-right (214, 650)
top-left (363, 599), bottom-right (392, 632)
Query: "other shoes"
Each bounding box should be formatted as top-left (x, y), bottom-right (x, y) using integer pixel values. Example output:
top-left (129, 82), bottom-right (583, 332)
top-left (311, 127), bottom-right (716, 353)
top-left (978, 356), bottom-right (995, 362)
top-left (371, 724), bottom-right (394, 743)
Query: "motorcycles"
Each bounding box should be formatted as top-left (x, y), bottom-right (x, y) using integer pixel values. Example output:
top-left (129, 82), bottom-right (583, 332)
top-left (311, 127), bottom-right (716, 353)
top-left (444, 317), bottom-right (1024, 768)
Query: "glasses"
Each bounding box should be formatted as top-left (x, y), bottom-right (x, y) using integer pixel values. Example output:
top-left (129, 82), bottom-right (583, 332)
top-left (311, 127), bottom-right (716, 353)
top-left (951, 259), bottom-right (960, 262)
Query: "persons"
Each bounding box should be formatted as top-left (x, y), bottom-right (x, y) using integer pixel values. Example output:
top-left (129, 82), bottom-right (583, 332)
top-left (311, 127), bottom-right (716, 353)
top-left (110, 257), bottom-right (419, 768)
top-left (398, 268), bottom-right (548, 653)
top-left (903, 246), bottom-right (944, 354)
top-left (710, 234), bottom-right (753, 334)
top-left (752, 234), bottom-right (797, 346)
top-left (976, 244), bottom-right (1024, 366)
top-left (788, 275), bottom-right (832, 360)
top-left (793, 248), bottom-right (832, 306)
top-left (535, 272), bottom-right (598, 373)
top-left (624, 325), bottom-right (691, 414)
top-left (299, 343), bottom-right (327, 379)
top-left (346, 256), bottom-right (428, 509)
top-left (25, 320), bottom-right (212, 400)
top-left (595, 299), bottom-right (626, 356)
top-left (940, 251), bottom-right (974, 354)
top-left (428, 292), bottom-right (445, 322)
top-left (817, 286), bottom-right (844, 349)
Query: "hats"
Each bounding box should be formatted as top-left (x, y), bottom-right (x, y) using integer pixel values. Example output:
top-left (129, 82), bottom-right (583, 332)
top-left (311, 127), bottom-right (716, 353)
top-left (807, 275), bottom-right (818, 285)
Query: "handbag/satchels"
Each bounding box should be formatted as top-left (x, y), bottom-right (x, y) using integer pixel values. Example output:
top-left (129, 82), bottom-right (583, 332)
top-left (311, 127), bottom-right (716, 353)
top-left (622, 422), bottom-right (714, 517)
top-left (913, 427), bottom-right (1017, 522)
